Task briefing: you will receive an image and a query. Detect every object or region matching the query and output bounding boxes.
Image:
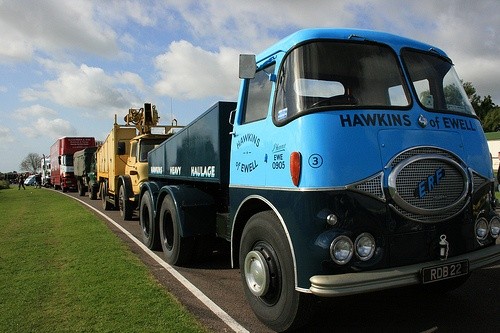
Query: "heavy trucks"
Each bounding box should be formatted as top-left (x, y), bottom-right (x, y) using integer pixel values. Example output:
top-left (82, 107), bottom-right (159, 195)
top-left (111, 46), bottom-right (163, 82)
top-left (139, 27), bottom-right (499, 332)
top-left (73, 147), bottom-right (96, 199)
top-left (50, 136), bottom-right (95, 191)
top-left (40, 154), bottom-right (50, 189)
top-left (95, 100), bottom-right (186, 221)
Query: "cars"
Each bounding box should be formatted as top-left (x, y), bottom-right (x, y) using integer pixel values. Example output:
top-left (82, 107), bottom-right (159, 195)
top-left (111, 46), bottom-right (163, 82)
top-left (9, 178), bottom-right (15, 184)
top-left (0, 177), bottom-right (8, 189)
top-left (24, 174), bottom-right (38, 186)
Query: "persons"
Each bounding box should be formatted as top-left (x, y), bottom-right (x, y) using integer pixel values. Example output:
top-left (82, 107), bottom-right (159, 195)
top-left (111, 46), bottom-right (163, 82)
top-left (18, 174), bottom-right (25, 190)
top-left (35, 173), bottom-right (41, 189)
top-left (497, 152), bottom-right (500, 192)
